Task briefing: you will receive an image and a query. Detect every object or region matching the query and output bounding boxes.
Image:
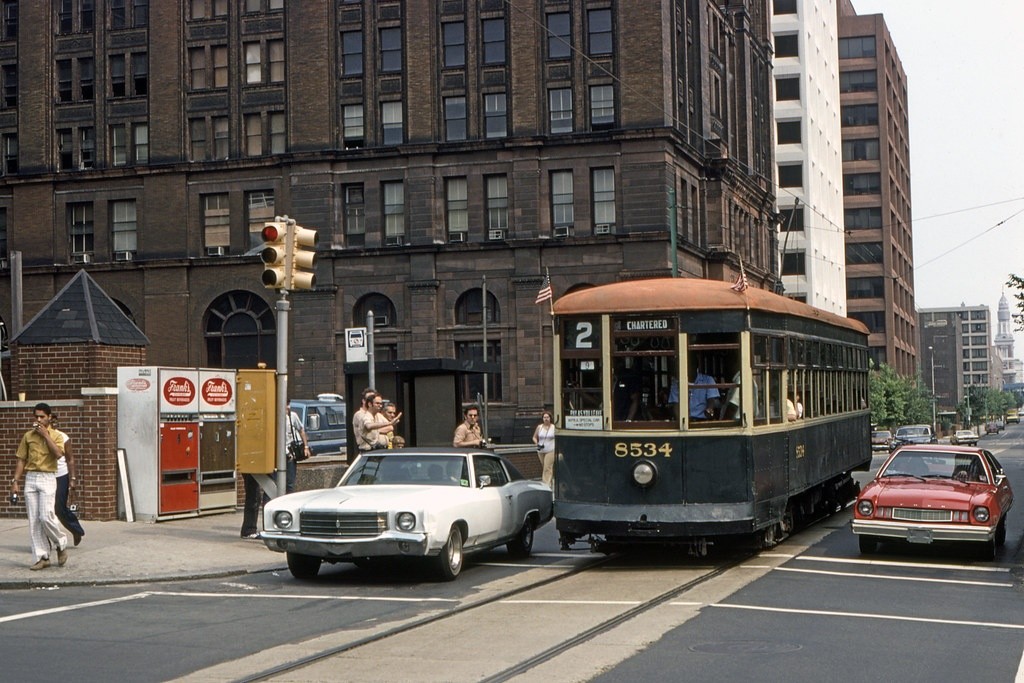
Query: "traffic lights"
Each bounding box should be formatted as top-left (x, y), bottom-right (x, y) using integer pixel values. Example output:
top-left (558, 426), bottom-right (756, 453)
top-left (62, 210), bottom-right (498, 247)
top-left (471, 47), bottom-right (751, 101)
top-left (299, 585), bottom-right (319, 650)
top-left (290, 225), bottom-right (319, 292)
top-left (260, 221), bottom-right (287, 289)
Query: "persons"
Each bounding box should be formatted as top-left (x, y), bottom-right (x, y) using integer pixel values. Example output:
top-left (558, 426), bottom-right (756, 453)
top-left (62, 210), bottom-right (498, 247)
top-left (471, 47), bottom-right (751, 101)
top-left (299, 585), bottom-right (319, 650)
top-left (380, 402), bottom-right (396, 449)
top-left (241, 473), bottom-right (263, 539)
top-left (286, 398), bottom-right (311, 495)
top-left (634, 344), bottom-right (663, 421)
top-left (797, 393), bottom-right (803, 420)
top-left (670, 354), bottom-right (719, 422)
top-left (358, 394), bottom-right (401, 452)
top-left (453, 408), bottom-right (484, 449)
top-left (596, 364), bottom-right (639, 422)
top-left (51, 415), bottom-right (85, 546)
top-left (533, 412), bottom-right (555, 487)
top-left (771, 385), bottom-right (796, 422)
top-left (11, 403), bottom-right (67, 570)
top-left (352, 389), bottom-right (394, 451)
top-left (720, 352), bottom-right (759, 422)
top-left (392, 436), bottom-right (406, 451)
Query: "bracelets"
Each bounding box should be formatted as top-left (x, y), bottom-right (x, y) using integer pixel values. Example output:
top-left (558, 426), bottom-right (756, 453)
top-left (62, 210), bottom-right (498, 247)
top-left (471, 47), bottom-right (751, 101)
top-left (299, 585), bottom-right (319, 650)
top-left (71, 476), bottom-right (76, 480)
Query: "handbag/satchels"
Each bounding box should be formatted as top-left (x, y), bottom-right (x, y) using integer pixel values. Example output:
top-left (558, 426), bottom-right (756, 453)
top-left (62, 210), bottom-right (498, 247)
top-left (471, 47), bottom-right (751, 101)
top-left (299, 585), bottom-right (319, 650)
top-left (293, 441), bottom-right (313, 462)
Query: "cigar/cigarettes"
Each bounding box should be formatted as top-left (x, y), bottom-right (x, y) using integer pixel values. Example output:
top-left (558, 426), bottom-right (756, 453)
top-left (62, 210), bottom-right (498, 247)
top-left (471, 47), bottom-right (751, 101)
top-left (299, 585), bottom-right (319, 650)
top-left (32, 424), bottom-right (39, 427)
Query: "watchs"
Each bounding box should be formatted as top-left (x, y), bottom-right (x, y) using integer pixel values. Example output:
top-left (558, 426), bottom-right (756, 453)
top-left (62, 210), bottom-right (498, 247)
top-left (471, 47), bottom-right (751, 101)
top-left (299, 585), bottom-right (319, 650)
top-left (11, 478), bottom-right (18, 484)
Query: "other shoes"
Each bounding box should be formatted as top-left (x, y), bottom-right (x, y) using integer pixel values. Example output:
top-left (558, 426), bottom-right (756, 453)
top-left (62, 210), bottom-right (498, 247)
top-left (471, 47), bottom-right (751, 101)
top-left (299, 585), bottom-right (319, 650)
top-left (73, 531), bottom-right (86, 546)
top-left (57, 546), bottom-right (68, 566)
top-left (30, 557), bottom-right (50, 570)
top-left (241, 533), bottom-right (263, 540)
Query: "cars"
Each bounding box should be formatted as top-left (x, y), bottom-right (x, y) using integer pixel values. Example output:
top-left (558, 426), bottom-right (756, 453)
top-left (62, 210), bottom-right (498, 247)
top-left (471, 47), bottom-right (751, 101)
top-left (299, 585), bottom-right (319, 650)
top-left (1018, 408), bottom-right (1024, 416)
top-left (1006, 410), bottom-right (1020, 424)
top-left (888, 423), bottom-right (938, 454)
top-left (871, 430), bottom-right (893, 452)
top-left (985, 420), bottom-right (1004, 435)
top-left (257, 443), bottom-right (555, 582)
top-left (849, 442), bottom-right (1016, 562)
top-left (950, 429), bottom-right (980, 446)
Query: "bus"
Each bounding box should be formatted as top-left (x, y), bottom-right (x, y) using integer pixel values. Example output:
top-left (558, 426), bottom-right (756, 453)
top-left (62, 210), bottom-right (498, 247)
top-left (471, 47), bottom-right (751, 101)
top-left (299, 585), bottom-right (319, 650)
top-left (546, 197), bottom-right (874, 557)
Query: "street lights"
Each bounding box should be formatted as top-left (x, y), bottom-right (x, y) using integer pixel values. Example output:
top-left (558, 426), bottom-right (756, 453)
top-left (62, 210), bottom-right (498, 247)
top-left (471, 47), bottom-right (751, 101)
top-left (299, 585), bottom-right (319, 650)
top-left (927, 345), bottom-right (938, 444)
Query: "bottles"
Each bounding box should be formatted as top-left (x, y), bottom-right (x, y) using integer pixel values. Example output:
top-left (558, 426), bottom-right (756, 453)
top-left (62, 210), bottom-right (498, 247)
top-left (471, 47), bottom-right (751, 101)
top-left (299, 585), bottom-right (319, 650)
top-left (11, 493), bottom-right (18, 504)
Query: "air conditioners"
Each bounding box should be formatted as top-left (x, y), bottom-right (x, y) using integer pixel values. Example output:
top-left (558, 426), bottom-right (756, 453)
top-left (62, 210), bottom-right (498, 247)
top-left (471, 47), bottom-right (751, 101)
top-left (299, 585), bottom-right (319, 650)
top-left (555, 228), bottom-right (573, 238)
top-left (449, 234), bottom-right (464, 243)
top-left (386, 236), bottom-right (403, 246)
top-left (374, 315), bottom-right (390, 326)
top-left (114, 251), bottom-right (132, 260)
top-left (74, 255), bottom-right (91, 263)
top-left (209, 247), bottom-right (225, 257)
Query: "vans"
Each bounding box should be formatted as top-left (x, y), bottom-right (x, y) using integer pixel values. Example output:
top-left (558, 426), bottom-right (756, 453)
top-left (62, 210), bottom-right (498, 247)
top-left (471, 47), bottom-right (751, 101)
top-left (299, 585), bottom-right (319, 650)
top-left (288, 393), bottom-right (347, 457)
